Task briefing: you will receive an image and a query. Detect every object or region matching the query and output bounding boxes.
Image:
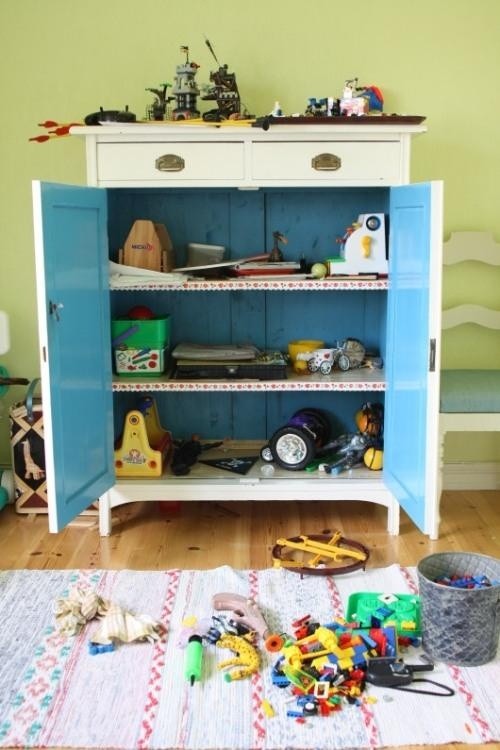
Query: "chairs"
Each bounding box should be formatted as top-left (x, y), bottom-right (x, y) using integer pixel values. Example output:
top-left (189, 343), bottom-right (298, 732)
top-left (438, 233), bottom-right (499, 555)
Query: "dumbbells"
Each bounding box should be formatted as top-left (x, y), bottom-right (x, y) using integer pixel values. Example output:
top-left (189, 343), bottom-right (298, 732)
top-left (272, 408), bottom-right (332, 471)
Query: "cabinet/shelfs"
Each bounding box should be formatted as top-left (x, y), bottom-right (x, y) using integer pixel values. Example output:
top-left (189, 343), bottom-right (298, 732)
top-left (30, 115), bottom-right (446, 544)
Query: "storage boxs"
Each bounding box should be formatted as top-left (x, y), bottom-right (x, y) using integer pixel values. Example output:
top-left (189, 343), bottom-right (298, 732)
top-left (111, 314), bottom-right (171, 378)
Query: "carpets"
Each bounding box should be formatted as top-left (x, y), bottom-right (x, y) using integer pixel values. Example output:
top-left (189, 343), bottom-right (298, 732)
top-left (1, 564), bottom-right (499, 747)
